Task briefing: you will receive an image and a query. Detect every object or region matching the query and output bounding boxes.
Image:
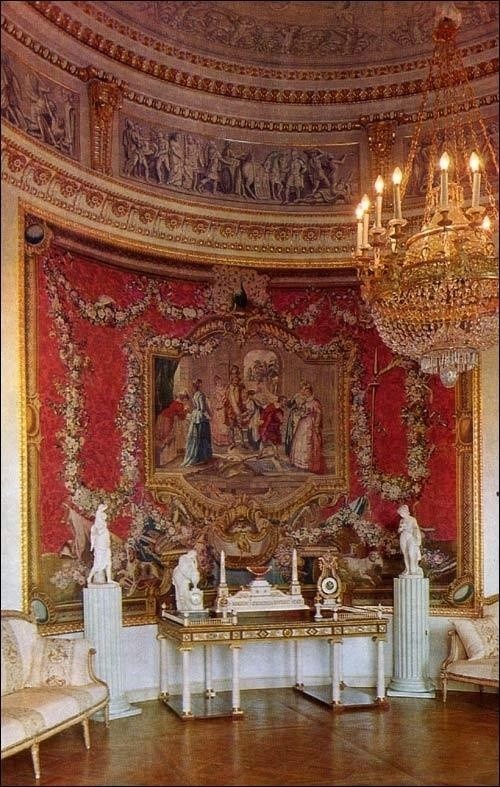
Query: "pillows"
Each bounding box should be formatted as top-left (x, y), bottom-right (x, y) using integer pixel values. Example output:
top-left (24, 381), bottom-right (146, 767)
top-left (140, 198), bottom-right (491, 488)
top-left (23, 636), bottom-right (92, 689)
top-left (447, 614), bottom-right (499, 661)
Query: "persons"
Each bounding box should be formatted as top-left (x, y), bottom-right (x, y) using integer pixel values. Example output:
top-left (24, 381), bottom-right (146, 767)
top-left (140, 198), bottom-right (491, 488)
top-left (396, 505), bottom-right (422, 575)
top-left (123, 119), bottom-right (348, 203)
top-left (172, 549), bottom-right (199, 611)
top-left (87, 504), bottom-right (115, 583)
top-left (154, 375), bottom-right (328, 474)
top-left (1, 55), bottom-right (76, 157)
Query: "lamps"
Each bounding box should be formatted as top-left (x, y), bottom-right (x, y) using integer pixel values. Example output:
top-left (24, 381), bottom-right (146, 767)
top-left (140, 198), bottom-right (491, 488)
top-left (352, 1), bottom-right (500, 389)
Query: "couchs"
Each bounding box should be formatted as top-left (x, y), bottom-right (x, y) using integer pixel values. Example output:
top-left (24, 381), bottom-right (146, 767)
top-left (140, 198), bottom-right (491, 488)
top-left (1, 610), bottom-right (112, 780)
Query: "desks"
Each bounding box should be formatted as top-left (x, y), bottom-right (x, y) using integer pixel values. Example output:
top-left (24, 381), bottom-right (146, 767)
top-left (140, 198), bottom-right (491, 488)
top-left (155, 605), bottom-right (390, 726)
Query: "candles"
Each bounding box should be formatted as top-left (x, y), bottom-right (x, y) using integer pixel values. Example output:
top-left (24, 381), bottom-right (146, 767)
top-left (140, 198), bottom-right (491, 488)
top-left (292, 548), bottom-right (298, 581)
top-left (219, 550), bottom-right (226, 583)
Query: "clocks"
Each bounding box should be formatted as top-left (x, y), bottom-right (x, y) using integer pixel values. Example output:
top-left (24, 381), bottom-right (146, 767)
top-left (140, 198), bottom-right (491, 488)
top-left (316, 548), bottom-right (342, 609)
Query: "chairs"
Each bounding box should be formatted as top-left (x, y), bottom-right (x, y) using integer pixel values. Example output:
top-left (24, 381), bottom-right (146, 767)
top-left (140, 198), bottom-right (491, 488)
top-left (439, 593), bottom-right (500, 703)
